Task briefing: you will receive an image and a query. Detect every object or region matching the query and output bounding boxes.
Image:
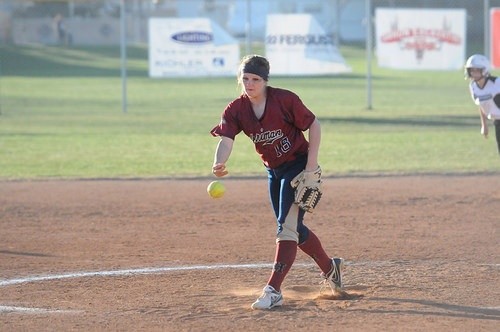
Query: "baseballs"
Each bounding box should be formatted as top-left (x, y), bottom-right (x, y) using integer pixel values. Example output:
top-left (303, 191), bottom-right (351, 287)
top-left (207, 181), bottom-right (226, 199)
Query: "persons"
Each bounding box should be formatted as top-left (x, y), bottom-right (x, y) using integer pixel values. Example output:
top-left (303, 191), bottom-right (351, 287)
top-left (211, 56), bottom-right (343, 310)
top-left (465, 54), bottom-right (500, 159)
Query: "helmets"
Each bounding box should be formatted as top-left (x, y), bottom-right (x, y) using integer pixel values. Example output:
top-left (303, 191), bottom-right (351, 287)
top-left (463, 55), bottom-right (490, 80)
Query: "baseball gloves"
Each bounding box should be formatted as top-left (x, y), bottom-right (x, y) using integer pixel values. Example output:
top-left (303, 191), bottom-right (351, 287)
top-left (290, 167), bottom-right (323, 213)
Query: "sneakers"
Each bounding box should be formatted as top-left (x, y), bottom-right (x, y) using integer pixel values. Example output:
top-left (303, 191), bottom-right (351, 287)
top-left (251, 284), bottom-right (283, 310)
top-left (320, 257), bottom-right (345, 297)
